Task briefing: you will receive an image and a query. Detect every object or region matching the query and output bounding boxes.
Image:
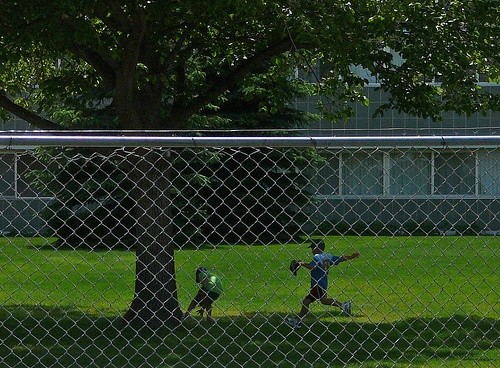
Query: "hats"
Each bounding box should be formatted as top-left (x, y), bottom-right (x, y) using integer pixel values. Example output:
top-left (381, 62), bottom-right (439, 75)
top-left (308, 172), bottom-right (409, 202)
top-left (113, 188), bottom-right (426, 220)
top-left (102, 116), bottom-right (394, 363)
top-left (196, 267), bottom-right (206, 282)
top-left (308, 239), bottom-right (325, 248)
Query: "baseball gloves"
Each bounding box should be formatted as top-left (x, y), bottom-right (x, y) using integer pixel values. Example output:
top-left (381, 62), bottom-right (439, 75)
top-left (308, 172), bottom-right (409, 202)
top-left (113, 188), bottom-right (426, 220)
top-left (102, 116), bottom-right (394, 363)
top-left (290, 260), bottom-right (302, 277)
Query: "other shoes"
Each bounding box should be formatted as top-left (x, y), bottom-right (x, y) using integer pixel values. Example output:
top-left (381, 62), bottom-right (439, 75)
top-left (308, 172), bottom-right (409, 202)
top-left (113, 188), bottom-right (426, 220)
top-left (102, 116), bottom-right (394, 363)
top-left (342, 302), bottom-right (351, 317)
top-left (183, 313), bottom-right (188, 318)
top-left (207, 316), bottom-right (212, 321)
top-left (286, 317), bottom-right (302, 329)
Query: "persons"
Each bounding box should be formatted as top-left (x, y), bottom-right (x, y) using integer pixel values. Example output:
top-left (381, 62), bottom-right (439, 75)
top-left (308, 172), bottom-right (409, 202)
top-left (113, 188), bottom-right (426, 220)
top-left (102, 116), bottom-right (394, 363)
top-left (285, 238), bottom-right (361, 329)
top-left (180, 266), bottom-right (224, 324)
